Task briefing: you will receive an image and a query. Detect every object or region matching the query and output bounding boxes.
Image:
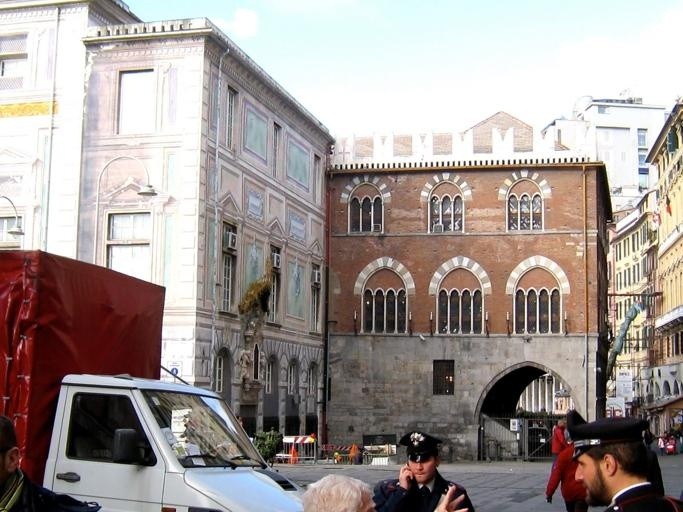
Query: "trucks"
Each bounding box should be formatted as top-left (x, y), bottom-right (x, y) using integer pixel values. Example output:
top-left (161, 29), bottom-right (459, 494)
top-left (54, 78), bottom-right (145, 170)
top-left (0, 251), bottom-right (306, 512)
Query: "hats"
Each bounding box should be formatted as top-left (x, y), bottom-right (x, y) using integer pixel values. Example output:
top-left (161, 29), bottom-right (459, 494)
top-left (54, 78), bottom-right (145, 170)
top-left (398, 431), bottom-right (442, 463)
top-left (570, 416), bottom-right (649, 460)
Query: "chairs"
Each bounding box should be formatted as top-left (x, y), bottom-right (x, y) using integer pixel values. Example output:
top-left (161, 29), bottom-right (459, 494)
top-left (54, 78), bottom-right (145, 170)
top-left (76, 418), bottom-right (148, 463)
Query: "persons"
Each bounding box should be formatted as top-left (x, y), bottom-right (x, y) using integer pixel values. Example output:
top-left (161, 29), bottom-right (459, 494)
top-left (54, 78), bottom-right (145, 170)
top-left (657, 430), bottom-right (683, 456)
top-left (303, 474), bottom-right (469, 512)
top-left (550, 419), bottom-right (567, 460)
top-left (571, 415), bottom-right (683, 512)
top-left (647, 451), bottom-right (664, 497)
top-left (644, 429), bottom-right (653, 449)
top-left (563, 428), bottom-right (571, 441)
top-left (546, 433), bottom-right (587, 512)
top-left (0, 416), bottom-right (97, 512)
top-left (372, 430), bottom-right (475, 512)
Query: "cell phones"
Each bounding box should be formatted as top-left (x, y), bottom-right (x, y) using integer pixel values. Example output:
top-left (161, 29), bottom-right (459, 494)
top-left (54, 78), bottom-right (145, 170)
top-left (408, 475), bottom-right (415, 489)
